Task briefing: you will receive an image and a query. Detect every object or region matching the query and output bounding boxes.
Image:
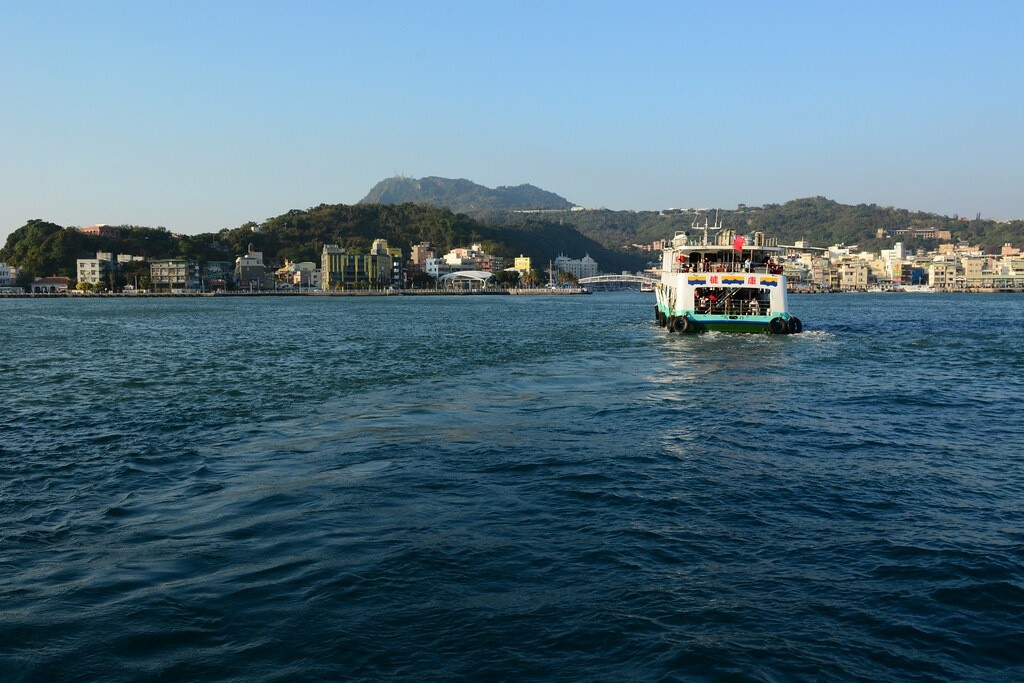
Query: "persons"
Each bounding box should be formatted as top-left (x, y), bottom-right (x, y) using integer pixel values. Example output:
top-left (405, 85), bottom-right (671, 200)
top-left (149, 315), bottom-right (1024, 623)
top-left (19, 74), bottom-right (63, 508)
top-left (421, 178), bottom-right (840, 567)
top-left (679, 254), bottom-right (711, 273)
top-left (735, 254), bottom-right (753, 272)
top-left (765, 259), bottom-right (777, 274)
top-left (741, 298), bottom-right (751, 315)
top-left (749, 297), bottom-right (761, 315)
top-left (695, 288), bottom-right (719, 314)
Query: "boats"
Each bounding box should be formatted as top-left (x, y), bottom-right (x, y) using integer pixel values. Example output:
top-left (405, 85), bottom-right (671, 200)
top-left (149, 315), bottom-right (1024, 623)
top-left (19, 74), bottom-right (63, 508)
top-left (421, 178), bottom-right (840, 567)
top-left (655, 227), bottom-right (802, 334)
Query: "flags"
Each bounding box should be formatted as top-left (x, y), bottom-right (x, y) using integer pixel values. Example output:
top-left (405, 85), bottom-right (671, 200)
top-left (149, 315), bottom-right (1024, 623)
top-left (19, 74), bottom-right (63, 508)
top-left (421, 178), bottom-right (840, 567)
top-left (733, 235), bottom-right (745, 253)
